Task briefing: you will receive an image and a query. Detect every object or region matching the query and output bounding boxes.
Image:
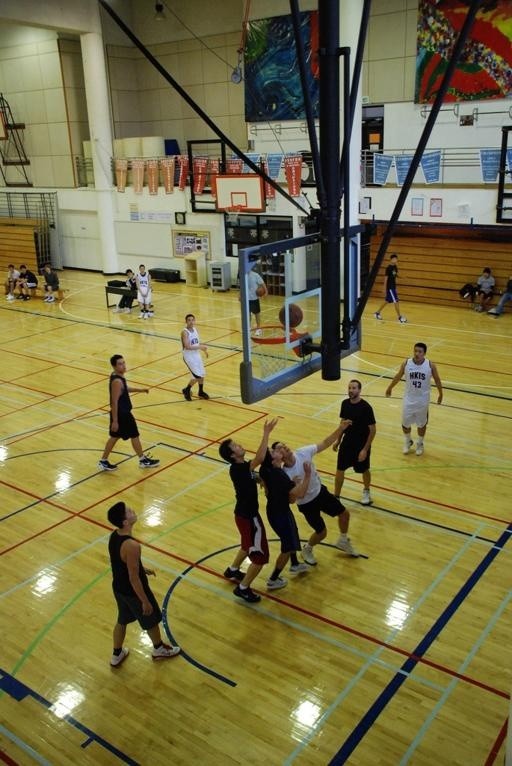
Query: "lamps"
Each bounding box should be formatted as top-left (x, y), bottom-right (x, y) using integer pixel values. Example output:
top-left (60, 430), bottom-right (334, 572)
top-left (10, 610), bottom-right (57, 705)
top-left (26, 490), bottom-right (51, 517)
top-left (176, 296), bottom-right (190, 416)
top-left (154, 0), bottom-right (167, 22)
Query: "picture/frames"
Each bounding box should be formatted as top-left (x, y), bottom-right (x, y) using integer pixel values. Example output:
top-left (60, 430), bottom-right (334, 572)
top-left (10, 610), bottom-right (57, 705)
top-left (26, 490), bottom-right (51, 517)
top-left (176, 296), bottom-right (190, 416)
top-left (175, 212), bottom-right (185, 224)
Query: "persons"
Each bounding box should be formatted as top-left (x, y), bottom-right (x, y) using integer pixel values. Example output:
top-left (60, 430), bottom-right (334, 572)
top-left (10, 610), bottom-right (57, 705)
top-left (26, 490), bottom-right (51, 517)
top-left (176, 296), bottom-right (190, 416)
top-left (476, 267), bottom-right (495, 312)
top-left (97, 355), bottom-right (161, 470)
top-left (136, 264), bottom-right (152, 318)
top-left (113, 269), bottom-right (137, 313)
top-left (260, 445), bottom-right (308, 589)
top-left (5, 264), bottom-right (59, 302)
top-left (385, 343), bottom-right (443, 456)
top-left (489, 277), bottom-right (512, 315)
top-left (181, 314), bottom-right (210, 402)
top-left (219, 417), bottom-right (279, 602)
top-left (238, 270), bottom-right (268, 337)
top-left (270, 418), bottom-right (359, 566)
top-left (105, 503), bottom-right (180, 669)
top-left (375, 253), bottom-right (408, 322)
top-left (333, 379), bottom-right (378, 505)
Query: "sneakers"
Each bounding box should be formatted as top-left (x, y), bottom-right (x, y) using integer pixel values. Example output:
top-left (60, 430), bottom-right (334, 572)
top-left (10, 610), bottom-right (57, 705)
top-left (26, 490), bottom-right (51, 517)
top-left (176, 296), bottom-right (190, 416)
top-left (6, 293), bottom-right (31, 301)
top-left (290, 563), bottom-right (307, 574)
top-left (336, 537), bottom-right (353, 552)
top-left (151, 642), bottom-right (180, 658)
top-left (98, 461), bottom-right (117, 470)
top-left (44, 296), bottom-right (55, 302)
top-left (110, 648), bottom-right (128, 665)
top-left (302, 545), bottom-right (317, 565)
top-left (224, 567), bottom-right (246, 580)
top-left (475, 304), bottom-right (500, 316)
top-left (183, 388), bottom-right (191, 400)
top-left (361, 488), bottom-right (369, 504)
top-left (416, 441), bottom-right (423, 455)
top-left (255, 329), bottom-right (262, 336)
top-left (267, 578), bottom-right (287, 589)
top-left (233, 584), bottom-right (260, 602)
top-left (374, 311), bottom-right (381, 320)
top-left (139, 457), bottom-right (160, 468)
top-left (198, 391), bottom-right (208, 399)
top-left (403, 440), bottom-right (413, 454)
top-left (398, 317), bottom-right (407, 322)
top-left (112, 309), bottom-right (149, 320)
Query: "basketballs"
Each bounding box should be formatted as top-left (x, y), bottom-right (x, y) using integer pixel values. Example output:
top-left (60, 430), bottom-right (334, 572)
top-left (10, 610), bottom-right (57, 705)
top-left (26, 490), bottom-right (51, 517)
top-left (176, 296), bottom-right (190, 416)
top-left (279, 303), bottom-right (302, 328)
top-left (256, 286), bottom-right (266, 297)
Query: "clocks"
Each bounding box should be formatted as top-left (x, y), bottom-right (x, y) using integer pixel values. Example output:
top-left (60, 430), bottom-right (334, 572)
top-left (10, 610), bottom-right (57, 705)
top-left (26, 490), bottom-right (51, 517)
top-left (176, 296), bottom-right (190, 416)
top-left (231, 72), bottom-right (242, 83)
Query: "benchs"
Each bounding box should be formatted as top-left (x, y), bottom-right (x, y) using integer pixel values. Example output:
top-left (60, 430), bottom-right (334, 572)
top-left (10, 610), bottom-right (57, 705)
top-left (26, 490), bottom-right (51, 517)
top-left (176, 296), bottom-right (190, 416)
top-left (148, 268), bottom-right (181, 283)
top-left (4, 283), bottom-right (69, 299)
top-left (368, 234), bottom-right (512, 314)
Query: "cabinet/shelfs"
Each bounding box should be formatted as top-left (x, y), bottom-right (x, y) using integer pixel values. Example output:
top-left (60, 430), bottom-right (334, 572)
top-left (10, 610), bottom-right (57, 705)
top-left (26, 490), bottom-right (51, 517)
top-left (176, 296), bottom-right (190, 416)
top-left (209, 261), bottom-right (231, 294)
top-left (185, 251), bottom-right (208, 287)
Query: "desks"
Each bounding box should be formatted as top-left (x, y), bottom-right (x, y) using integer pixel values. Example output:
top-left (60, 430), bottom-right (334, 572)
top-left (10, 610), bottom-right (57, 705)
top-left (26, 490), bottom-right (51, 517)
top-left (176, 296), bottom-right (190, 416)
top-left (104, 285), bottom-right (154, 308)
